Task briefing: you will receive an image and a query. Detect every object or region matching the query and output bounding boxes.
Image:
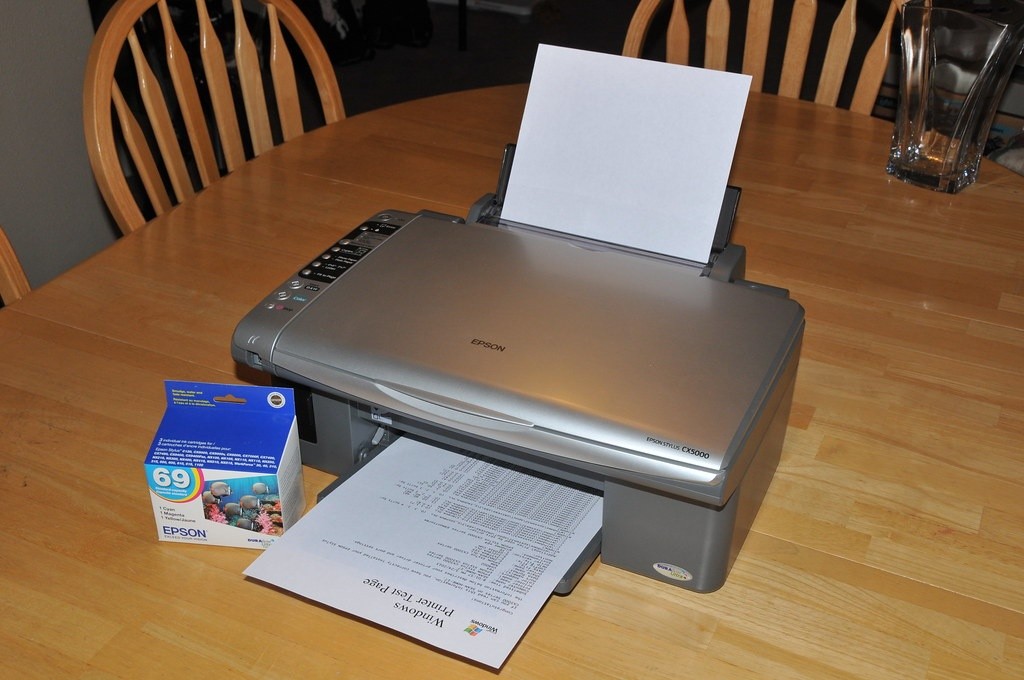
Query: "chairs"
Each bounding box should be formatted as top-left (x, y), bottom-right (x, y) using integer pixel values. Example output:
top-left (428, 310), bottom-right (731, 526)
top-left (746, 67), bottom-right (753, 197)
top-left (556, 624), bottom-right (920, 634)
top-left (621, 0), bottom-right (931, 129)
top-left (83, 0), bottom-right (346, 234)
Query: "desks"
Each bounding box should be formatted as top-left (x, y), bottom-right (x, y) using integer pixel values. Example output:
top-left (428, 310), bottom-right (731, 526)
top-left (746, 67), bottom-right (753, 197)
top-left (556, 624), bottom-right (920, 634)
top-left (0, 81), bottom-right (1024, 680)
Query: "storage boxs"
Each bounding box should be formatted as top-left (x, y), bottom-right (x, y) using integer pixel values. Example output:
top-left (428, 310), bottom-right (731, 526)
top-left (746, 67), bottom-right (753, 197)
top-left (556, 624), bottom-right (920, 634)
top-left (144, 380), bottom-right (309, 550)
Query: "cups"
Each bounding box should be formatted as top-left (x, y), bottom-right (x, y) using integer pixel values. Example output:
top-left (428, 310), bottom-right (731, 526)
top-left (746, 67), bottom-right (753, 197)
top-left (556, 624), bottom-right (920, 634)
top-left (884, 0), bottom-right (1024, 194)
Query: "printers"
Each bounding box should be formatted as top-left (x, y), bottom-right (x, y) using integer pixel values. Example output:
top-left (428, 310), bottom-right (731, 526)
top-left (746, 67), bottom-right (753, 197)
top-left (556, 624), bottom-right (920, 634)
top-left (230, 144), bottom-right (804, 595)
